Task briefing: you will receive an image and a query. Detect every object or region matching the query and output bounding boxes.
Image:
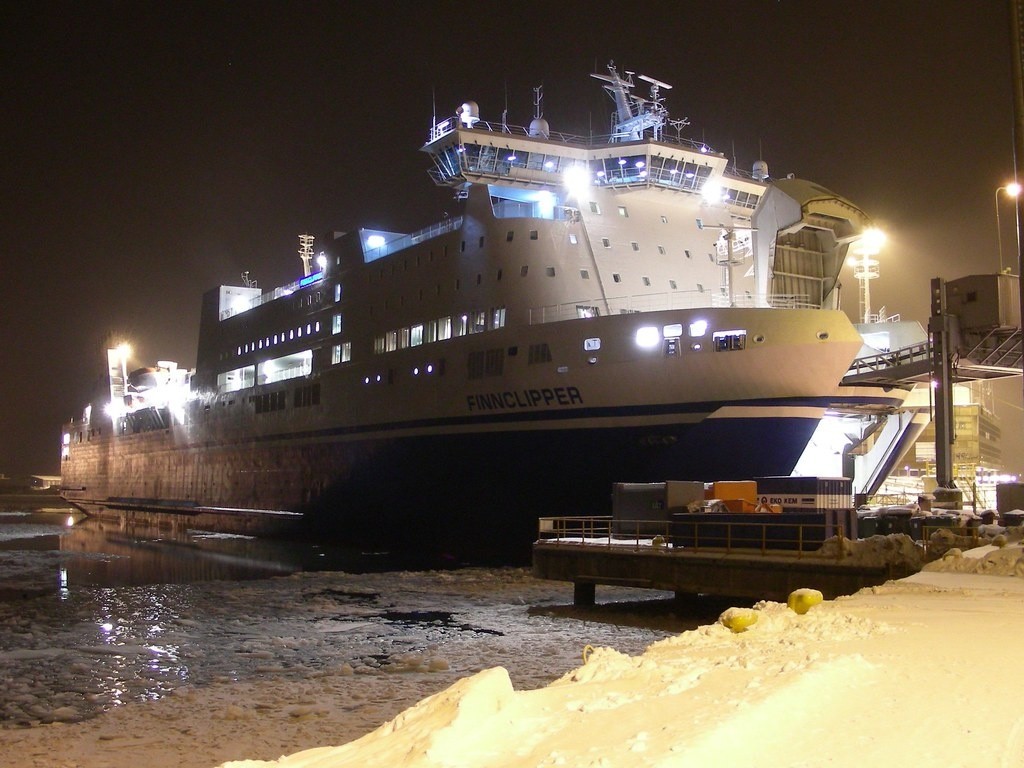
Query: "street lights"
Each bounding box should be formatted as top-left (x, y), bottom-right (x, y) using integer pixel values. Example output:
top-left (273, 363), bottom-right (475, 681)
top-left (847, 225), bottom-right (887, 326)
top-left (994, 182), bottom-right (1023, 273)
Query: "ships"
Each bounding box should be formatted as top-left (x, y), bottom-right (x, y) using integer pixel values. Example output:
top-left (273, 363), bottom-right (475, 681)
top-left (59, 58), bottom-right (866, 556)
